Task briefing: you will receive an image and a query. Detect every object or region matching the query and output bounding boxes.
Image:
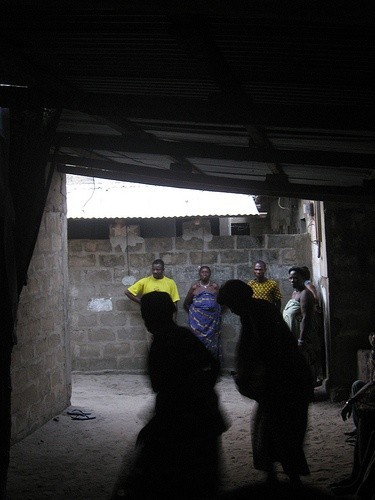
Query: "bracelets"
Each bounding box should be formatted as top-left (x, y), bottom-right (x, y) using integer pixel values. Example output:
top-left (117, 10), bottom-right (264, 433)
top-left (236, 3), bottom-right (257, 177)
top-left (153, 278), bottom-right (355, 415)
top-left (298, 339), bottom-right (304, 343)
top-left (346, 401), bottom-right (351, 407)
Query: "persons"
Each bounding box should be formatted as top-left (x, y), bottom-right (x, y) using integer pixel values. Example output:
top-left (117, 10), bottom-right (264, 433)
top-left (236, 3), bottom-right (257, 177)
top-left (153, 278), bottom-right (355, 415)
top-left (183, 266), bottom-right (223, 382)
top-left (129, 291), bottom-right (230, 500)
top-left (341, 328), bottom-right (375, 443)
top-left (217, 279), bottom-right (316, 483)
top-left (246, 260), bottom-right (282, 310)
top-left (282, 266), bottom-right (324, 387)
top-left (124, 259), bottom-right (180, 375)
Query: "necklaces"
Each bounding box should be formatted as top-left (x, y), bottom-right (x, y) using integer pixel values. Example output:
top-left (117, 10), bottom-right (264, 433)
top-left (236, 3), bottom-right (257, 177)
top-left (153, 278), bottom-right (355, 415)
top-left (200, 280), bottom-right (211, 288)
